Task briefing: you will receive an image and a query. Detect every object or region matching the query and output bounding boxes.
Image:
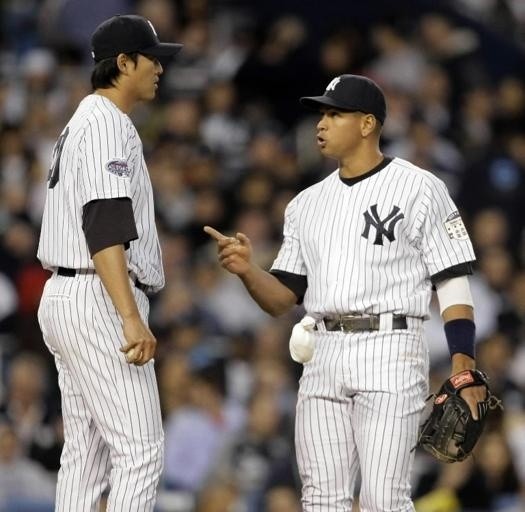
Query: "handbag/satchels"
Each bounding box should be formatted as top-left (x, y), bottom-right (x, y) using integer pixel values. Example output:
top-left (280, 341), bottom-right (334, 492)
top-left (443, 318), bottom-right (477, 361)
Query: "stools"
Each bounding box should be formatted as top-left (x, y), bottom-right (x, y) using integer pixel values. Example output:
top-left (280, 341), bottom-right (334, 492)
top-left (56, 265), bottom-right (148, 294)
top-left (312, 313), bottom-right (405, 333)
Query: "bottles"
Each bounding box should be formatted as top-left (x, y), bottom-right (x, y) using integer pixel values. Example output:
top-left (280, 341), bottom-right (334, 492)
top-left (299, 72), bottom-right (386, 124)
top-left (89, 13), bottom-right (185, 62)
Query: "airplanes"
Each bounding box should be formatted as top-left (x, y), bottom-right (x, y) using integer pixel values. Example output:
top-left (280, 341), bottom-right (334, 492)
top-left (410, 369), bottom-right (504, 464)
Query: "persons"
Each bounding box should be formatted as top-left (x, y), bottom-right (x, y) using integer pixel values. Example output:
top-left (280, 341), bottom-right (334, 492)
top-left (36, 14), bottom-right (184, 510)
top-left (208, 73), bottom-right (489, 510)
